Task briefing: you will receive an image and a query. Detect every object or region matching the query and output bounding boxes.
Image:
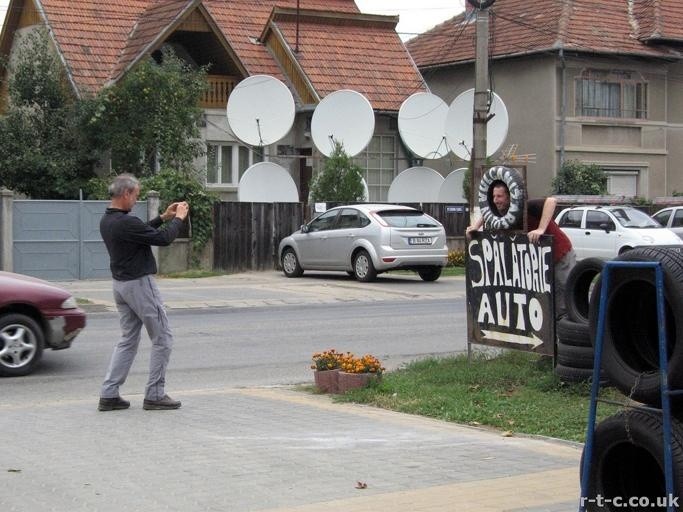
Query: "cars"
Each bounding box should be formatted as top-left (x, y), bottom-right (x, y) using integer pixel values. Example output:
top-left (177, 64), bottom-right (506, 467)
top-left (0, 270), bottom-right (88, 377)
top-left (277, 200), bottom-right (449, 284)
top-left (550, 202), bottom-right (683, 260)
top-left (648, 206), bottom-right (683, 239)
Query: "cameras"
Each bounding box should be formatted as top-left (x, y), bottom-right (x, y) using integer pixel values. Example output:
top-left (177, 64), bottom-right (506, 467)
top-left (173, 203), bottom-right (180, 212)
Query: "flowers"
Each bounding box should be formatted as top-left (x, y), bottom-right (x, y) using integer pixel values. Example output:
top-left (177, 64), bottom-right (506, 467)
top-left (311, 348), bottom-right (386, 373)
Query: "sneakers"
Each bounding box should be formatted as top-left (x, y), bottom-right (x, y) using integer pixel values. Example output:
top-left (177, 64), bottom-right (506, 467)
top-left (142, 394), bottom-right (181, 410)
top-left (98, 398), bottom-right (130, 411)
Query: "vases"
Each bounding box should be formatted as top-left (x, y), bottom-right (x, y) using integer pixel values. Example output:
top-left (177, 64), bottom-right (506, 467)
top-left (338, 370), bottom-right (377, 394)
top-left (314, 368), bottom-right (340, 392)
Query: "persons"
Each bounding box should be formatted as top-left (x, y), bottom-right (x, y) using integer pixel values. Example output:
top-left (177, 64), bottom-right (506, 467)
top-left (465, 181), bottom-right (577, 368)
top-left (98, 173), bottom-right (191, 410)
